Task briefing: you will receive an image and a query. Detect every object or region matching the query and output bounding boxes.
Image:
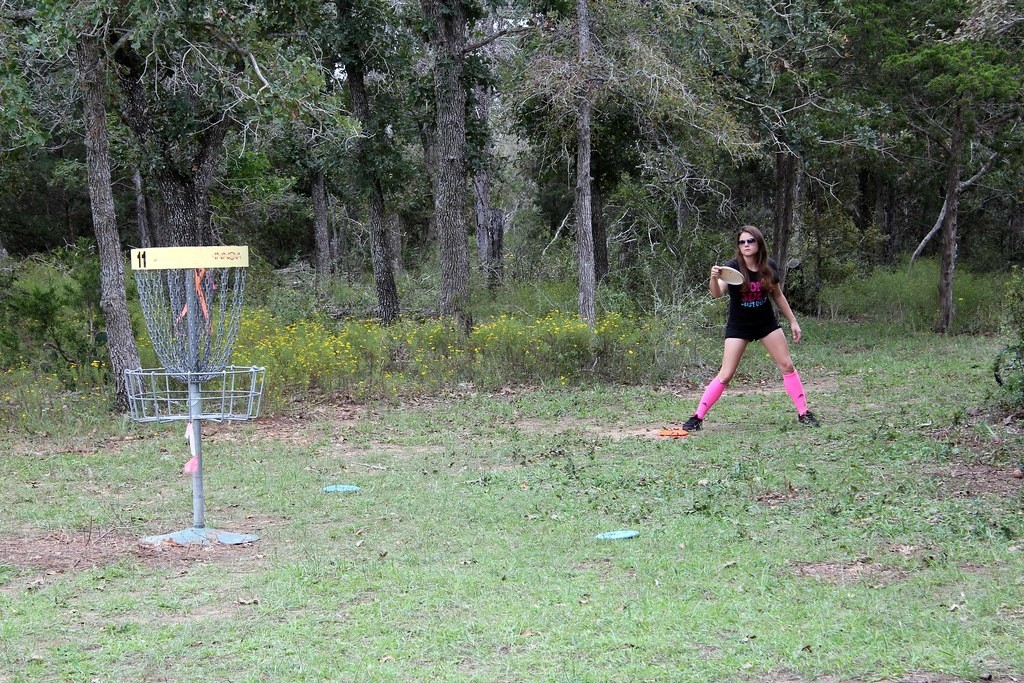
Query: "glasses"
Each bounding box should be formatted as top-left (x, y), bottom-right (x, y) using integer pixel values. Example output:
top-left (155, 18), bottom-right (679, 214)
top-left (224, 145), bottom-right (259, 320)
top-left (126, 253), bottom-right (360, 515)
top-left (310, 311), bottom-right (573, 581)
top-left (738, 238), bottom-right (756, 245)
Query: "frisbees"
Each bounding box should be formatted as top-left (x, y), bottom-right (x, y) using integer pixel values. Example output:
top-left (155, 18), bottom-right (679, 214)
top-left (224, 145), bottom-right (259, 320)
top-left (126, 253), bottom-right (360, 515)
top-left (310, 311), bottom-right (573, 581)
top-left (718, 265), bottom-right (745, 286)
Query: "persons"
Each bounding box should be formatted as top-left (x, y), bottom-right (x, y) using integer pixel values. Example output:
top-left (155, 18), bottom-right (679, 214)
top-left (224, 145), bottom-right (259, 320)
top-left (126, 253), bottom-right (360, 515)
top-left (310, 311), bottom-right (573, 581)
top-left (682, 225), bottom-right (819, 431)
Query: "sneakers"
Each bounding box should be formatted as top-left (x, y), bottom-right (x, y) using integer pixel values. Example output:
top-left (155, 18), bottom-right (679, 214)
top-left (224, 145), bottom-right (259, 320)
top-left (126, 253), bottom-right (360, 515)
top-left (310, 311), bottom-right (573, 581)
top-left (682, 414), bottom-right (703, 433)
top-left (798, 410), bottom-right (819, 429)
